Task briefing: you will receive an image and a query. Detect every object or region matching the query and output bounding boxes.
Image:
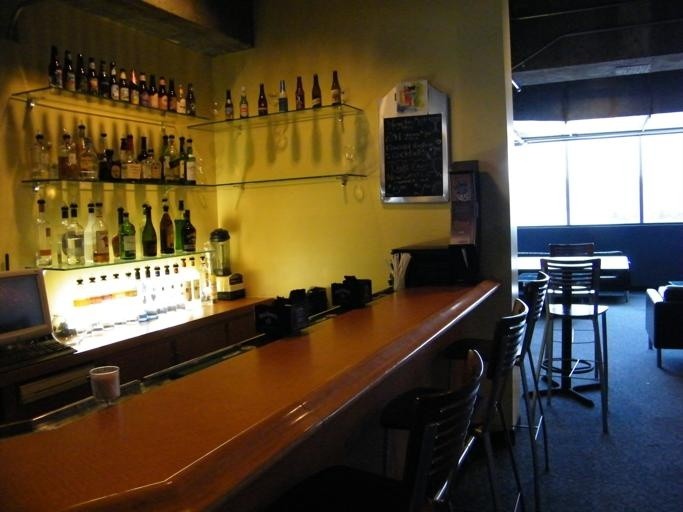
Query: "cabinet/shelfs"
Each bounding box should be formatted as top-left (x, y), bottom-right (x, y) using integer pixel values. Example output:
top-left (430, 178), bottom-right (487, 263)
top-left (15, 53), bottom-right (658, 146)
top-left (5, 84), bottom-right (370, 276)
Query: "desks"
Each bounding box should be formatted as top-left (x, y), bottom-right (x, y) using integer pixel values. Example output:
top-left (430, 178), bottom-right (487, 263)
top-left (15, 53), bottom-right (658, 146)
top-left (514, 251), bottom-right (634, 410)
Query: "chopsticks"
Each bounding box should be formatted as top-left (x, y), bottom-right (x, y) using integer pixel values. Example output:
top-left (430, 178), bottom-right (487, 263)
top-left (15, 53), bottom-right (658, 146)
top-left (384, 252), bottom-right (412, 290)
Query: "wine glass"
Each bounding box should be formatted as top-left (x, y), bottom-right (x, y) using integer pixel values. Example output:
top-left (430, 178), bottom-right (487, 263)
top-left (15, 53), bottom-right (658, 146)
top-left (343, 144), bottom-right (356, 174)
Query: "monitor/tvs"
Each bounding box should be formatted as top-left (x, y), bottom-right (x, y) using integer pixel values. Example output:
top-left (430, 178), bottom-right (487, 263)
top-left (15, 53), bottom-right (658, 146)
top-left (0, 268), bottom-right (53, 352)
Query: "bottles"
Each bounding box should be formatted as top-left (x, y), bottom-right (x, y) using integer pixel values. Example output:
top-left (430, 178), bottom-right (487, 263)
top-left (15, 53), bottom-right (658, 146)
top-left (73, 256), bottom-right (208, 335)
top-left (27, 135), bottom-right (51, 179)
top-left (54, 198), bottom-right (195, 262)
top-left (278, 80), bottom-right (287, 112)
top-left (238, 86), bottom-right (247, 118)
top-left (224, 89), bottom-right (234, 120)
top-left (57, 122), bottom-right (197, 184)
top-left (312, 73), bottom-right (320, 107)
top-left (294, 77), bottom-right (304, 109)
top-left (258, 80), bottom-right (266, 114)
top-left (33, 199), bottom-right (51, 266)
top-left (330, 71), bottom-right (341, 104)
top-left (47, 45), bottom-right (196, 118)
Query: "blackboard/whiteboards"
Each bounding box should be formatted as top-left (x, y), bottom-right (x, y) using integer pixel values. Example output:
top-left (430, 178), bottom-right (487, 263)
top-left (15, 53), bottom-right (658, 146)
top-left (379, 80), bottom-right (450, 204)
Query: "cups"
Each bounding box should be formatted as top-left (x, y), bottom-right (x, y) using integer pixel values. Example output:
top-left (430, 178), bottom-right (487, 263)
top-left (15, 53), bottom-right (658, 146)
top-left (88, 365), bottom-right (120, 401)
top-left (50, 315), bottom-right (80, 345)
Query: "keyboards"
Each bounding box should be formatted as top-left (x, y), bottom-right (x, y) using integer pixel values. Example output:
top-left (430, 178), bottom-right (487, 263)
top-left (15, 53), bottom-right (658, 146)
top-left (0, 341), bottom-right (77, 374)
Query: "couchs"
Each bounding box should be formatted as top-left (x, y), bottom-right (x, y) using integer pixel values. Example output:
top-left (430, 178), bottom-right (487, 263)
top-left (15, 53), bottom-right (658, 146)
top-left (642, 281), bottom-right (683, 368)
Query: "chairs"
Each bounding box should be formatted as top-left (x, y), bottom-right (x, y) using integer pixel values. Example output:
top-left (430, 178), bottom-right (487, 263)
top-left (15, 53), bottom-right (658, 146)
top-left (547, 240), bottom-right (599, 263)
top-left (442, 268), bottom-right (551, 455)
top-left (265, 343), bottom-right (487, 511)
top-left (537, 258), bottom-right (611, 437)
top-left (375, 296), bottom-right (533, 510)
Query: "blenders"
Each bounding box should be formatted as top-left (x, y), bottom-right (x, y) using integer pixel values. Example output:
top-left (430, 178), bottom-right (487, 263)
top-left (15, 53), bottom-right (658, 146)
top-left (209, 228), bottom-right (245, 299)
